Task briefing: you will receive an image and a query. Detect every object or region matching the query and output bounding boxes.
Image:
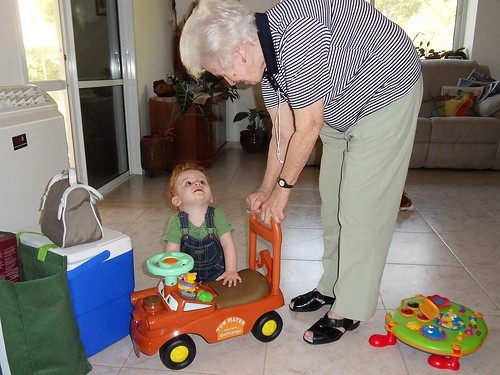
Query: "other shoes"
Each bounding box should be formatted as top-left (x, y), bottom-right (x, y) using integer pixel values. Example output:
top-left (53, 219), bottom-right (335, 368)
top-left (399, 192), bottom-right (413, 210)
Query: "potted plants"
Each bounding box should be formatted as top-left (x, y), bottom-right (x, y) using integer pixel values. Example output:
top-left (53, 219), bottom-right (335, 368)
top-left (141, 72), bottom-right (240, 177)
top-left (443, 47), bottom-right (467, 59)
top-left (233, 109), bottom-right (270, 153)
top-left (424, 41), bottom-right (442, 60)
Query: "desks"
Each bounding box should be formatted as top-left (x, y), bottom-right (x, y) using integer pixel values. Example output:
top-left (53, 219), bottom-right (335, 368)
top-left (150, 92), bottom-right (228, 169)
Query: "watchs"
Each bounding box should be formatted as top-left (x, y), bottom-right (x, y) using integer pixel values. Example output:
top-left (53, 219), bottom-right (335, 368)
top-left (277, 177), bottom-right (294, 188)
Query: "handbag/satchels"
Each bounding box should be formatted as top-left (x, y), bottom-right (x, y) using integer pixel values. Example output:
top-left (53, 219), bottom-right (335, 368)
top-left (430, 91), bottom-right (475, 117)
top-left (40, 168), bottom-right (104, 248)
top-left (0, 231), bottom-right (93, 375)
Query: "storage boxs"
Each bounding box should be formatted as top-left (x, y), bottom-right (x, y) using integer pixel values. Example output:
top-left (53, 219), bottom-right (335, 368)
top-left (17, 223), bottom-right (136, 358)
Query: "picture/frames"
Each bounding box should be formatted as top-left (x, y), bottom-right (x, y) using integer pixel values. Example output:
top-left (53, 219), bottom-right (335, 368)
top-left (95, 0), bottom-right (106, 16)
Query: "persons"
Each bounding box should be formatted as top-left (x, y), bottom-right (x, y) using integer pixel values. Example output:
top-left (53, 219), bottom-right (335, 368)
top-left (162, 160), bottom-right (242, 287)
top-left (179, 0), bottom-right (424, 345)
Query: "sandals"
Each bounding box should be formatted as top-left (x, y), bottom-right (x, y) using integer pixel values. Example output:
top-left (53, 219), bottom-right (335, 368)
top-left (302, 312), bottom-right (360, 344)
top-left (289, 287), bottom-right (336, 312)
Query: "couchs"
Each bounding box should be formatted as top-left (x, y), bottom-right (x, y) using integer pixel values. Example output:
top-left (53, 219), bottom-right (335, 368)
top-left (306, 59), bottom-right (500, 170)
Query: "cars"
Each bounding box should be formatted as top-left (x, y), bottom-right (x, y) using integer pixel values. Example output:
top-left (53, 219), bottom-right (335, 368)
top-left (129, 209), bottom-right (283, 370)
